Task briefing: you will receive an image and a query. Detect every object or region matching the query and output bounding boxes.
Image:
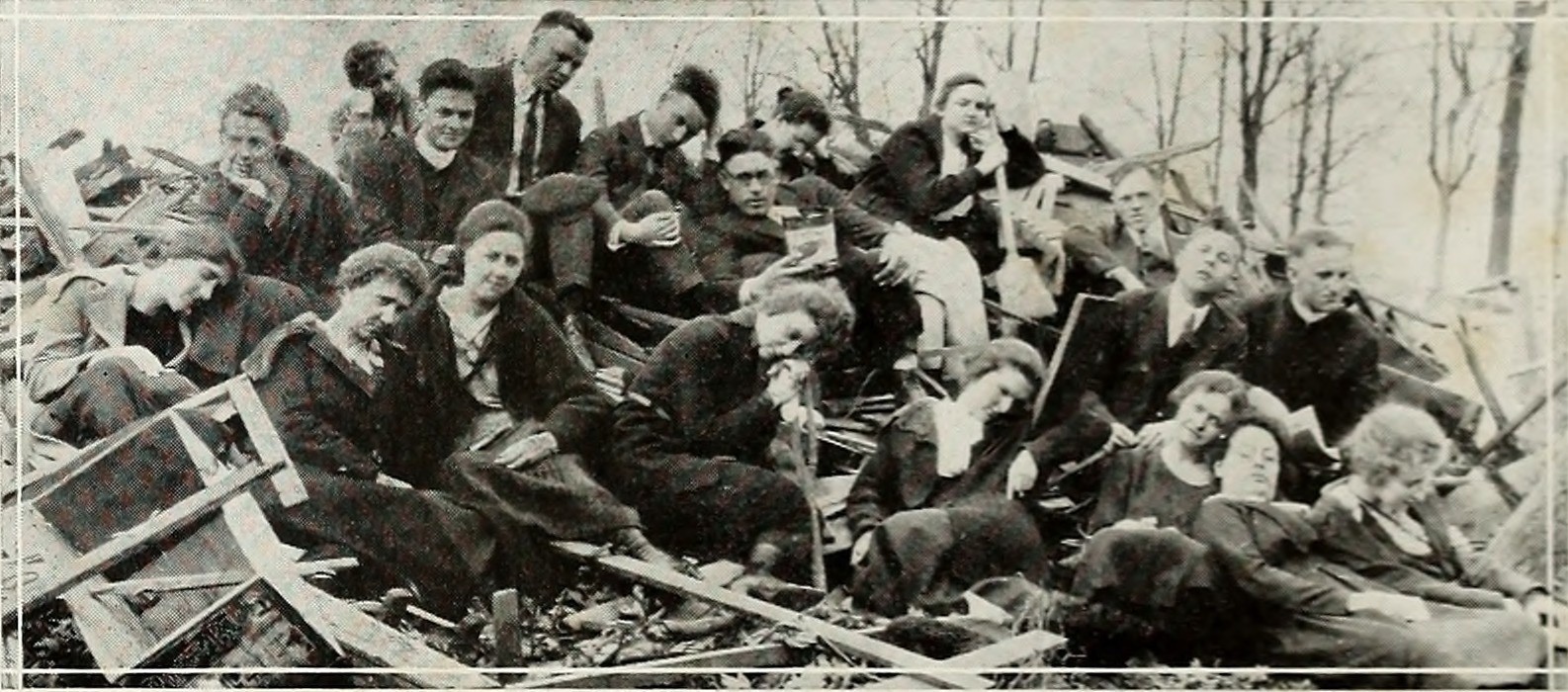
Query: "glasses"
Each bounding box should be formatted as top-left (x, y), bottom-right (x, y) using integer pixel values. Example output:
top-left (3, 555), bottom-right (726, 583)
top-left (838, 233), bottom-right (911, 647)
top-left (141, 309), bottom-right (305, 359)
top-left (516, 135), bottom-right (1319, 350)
top-left (720, 166), bottom-right (774, 188)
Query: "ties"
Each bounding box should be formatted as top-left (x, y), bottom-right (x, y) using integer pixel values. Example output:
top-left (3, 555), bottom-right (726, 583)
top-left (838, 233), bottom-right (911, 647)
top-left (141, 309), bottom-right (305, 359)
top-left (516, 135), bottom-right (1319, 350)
top-left (519, 89), bottom-right (541, 190)
top-left (1140, 231), bottom-right (1150, 249)
top-left (1173, 315), bottom-right (1197, 356)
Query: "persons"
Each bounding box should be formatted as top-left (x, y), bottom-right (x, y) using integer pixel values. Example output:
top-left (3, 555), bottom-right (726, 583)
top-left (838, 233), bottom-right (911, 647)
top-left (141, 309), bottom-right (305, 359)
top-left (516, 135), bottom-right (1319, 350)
top-left (409, 200), bottom-right (679, 587)
top-left (343, 58), bottom-right (502, 247)
top-left (1237, 225), bottom-right (1377, 503)
top-left (1063, 159), bottom-right (1558, 692)
top-left (695, 128), bottom-right (926, 392)
top-left (599, 283), bottom-right (853, 603)
top-left (331, 41), bottom-right (417, 185)
top-left (574, 66), bottom-right (724, 312)
top-left (22, 225), bottom-right (242, 448)
top-left (169, 85), bottom-right (354, 384)
top-left (236, 242), bottom-right (498, 606)
top-left (473, 8), bottom-right (593, 372)
top-left (848, 75), bottom-right (1044, 381)
top-left (847, 336), bottom-right (1109, 609)
top-left (699, 85), bottom-right (854, 208)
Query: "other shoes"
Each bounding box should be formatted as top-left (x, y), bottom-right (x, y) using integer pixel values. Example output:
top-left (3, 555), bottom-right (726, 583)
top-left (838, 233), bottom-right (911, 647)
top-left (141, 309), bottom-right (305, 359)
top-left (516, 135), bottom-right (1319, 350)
top-left (895, 369), bottom-right (930, 409)
top-left (640, 548), bottom-right (675, 573)
